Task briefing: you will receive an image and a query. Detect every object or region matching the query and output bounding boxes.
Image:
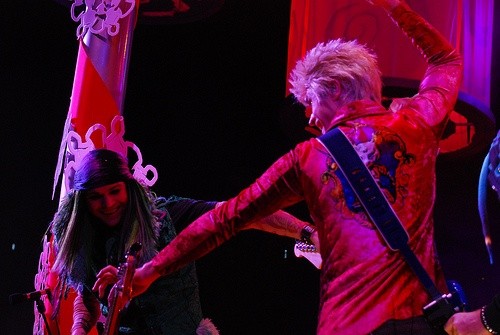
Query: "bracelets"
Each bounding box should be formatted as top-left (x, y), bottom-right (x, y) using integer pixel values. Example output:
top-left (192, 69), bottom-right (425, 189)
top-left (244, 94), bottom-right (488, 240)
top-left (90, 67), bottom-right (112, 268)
top-left (481, 304), bottom-right (498, 335)
top-left (71, 323), bottom-right (84, 332)
top-left (300, 223), bottom-right (318, 245)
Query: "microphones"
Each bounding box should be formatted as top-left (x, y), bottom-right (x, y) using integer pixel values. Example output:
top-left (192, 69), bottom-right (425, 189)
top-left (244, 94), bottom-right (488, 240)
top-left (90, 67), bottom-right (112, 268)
top-left (10, 288), bottom-right (49, 305)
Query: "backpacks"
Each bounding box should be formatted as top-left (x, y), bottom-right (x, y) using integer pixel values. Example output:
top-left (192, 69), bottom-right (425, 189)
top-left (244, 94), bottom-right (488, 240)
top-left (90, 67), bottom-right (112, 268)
top-left (424, 290), bottom-right (462, 335)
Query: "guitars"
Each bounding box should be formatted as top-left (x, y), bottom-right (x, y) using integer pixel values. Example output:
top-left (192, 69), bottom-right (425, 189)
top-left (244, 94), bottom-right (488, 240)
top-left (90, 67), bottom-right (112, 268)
top-left (102, 241), bottom-right (142, 335)
top-left (294, 242), bottom-right (323, 270)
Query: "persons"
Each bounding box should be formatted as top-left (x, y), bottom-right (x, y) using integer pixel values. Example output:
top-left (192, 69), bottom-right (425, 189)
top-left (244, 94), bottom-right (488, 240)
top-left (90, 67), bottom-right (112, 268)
top-left (53, 149), bottom-right (322, 335)
top-left (444, 290), bottom-right (500, 335)
top-left (92, 0), bottom-right (465, 335)
